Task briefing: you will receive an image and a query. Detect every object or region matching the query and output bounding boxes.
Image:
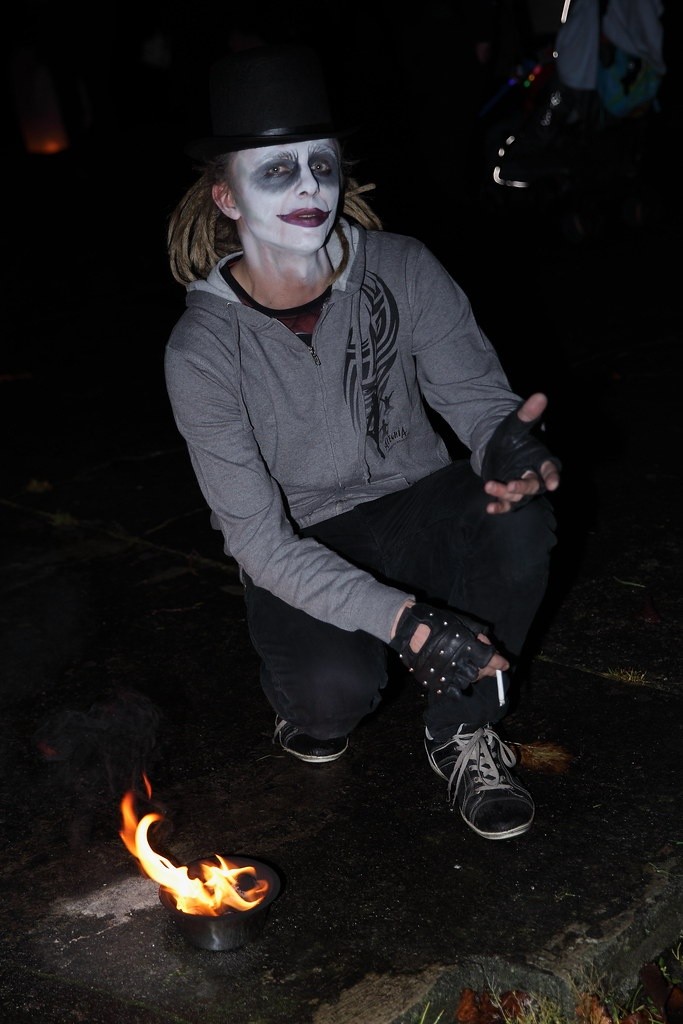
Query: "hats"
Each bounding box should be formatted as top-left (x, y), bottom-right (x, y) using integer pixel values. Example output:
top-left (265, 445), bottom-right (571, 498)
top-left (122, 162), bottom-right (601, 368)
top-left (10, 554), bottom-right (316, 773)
top-left (206, 41), bottom-right (361, 156)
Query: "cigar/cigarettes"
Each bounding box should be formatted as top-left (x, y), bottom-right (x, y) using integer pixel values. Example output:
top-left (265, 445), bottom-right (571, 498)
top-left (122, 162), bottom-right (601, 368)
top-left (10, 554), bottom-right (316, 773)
top-left (496, 668), bottom-right (506, 707)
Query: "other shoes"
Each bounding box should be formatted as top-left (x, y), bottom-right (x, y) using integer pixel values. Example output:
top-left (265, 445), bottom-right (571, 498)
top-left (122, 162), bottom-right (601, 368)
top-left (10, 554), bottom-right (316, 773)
top-left (423, 723), bottom-right (536, 842)
top-left (274, 714), bottom-right (349, 763)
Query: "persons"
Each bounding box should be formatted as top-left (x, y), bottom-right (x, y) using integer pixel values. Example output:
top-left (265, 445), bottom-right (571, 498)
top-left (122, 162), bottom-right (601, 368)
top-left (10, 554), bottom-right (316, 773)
top-left (165, 82), bottom-right (563, 843)
